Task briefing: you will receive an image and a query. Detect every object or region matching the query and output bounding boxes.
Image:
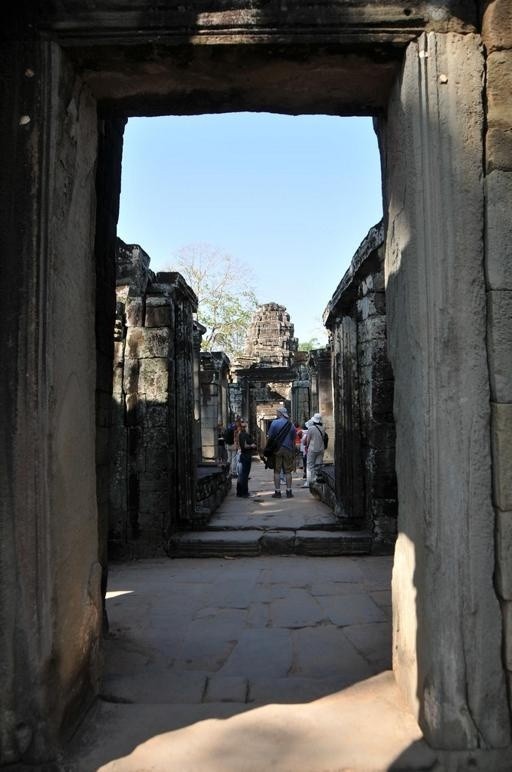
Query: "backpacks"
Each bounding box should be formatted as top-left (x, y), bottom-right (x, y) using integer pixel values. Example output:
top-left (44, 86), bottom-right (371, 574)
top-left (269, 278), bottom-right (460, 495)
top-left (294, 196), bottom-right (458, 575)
top-left (223, 426), bottom-right (237, 445)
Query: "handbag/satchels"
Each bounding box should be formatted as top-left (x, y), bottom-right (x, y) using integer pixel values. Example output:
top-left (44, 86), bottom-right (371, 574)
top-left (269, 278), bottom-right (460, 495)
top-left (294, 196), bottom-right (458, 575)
top-left (323, 433), bottom-right (328, 449)
top-left (263, 437), bottom-right (280, 457)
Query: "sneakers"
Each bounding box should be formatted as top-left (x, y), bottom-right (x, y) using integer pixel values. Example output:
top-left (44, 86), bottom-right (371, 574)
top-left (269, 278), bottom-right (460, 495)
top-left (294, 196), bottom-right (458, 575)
top-left (286, 489), bottom-right (294, 498)
top-left (236, 493), bottom-right (251, 498)
top-left (272, 491), bottom-right (281, 498)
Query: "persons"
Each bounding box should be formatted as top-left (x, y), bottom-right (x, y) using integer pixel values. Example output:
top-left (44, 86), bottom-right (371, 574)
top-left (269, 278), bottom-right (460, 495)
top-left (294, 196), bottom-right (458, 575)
top-left (223, 408), bottom-right (330, 498)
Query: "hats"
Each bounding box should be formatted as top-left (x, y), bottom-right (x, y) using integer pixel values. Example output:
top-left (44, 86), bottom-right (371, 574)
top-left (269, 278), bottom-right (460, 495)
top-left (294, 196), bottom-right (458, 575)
top-left (311, 413), bottom-right (323, 423)
top-left (276, 406), bottom-right (289, 418)
